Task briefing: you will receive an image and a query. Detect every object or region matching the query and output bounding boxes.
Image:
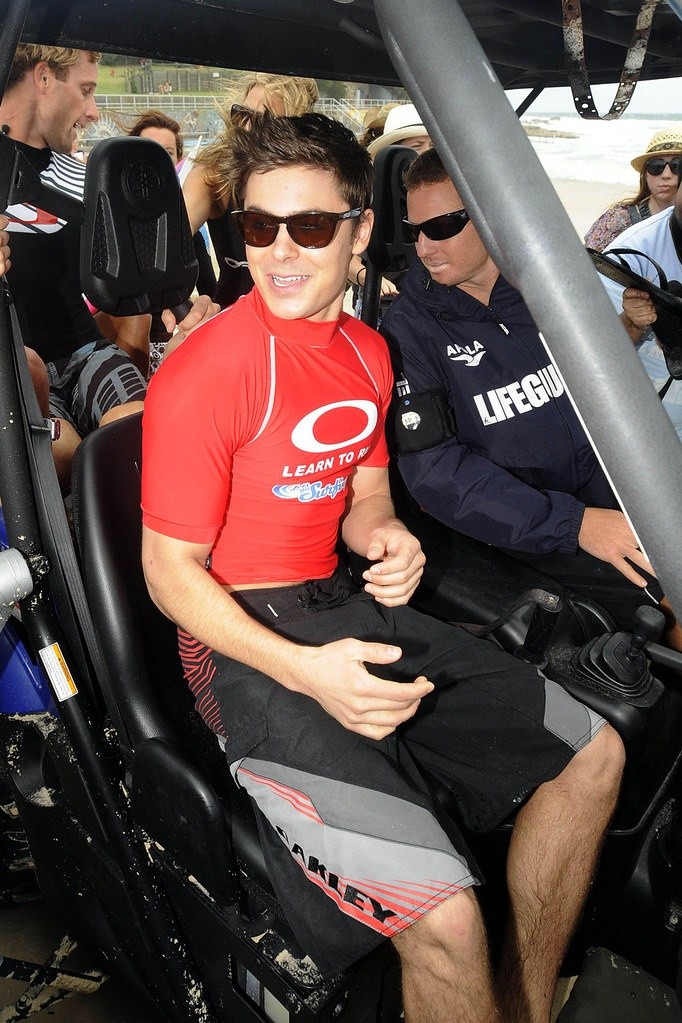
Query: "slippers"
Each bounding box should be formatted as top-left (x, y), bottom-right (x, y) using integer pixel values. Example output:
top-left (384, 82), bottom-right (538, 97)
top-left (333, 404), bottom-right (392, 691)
top-left (586, 247), bottom-right (682, 316)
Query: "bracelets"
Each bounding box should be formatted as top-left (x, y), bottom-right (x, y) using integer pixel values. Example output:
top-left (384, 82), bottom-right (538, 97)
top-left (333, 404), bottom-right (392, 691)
top-left (356, 268), bottom-right (367, 287)
top-left (629, 318), bottom-right (640, 330)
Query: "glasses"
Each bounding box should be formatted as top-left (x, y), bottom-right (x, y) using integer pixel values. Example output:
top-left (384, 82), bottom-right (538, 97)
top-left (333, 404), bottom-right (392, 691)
top-left (645, 157), bottom-right (682, 176)
top-left (230, 104), bottom-right (251, 128)
top-left (231, 205), bottom-right (365, 249)
top-left (400, 209), bottom-right (471, 245)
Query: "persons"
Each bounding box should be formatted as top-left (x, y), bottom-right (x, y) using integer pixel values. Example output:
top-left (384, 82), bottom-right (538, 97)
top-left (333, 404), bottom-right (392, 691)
top-left (348, 100), bottom-right (682, 652)
top-left (141, 106), bottom-right (627, 1022)
top-left (0, 41), bottom-right (318, 502)
top-left (595, 184), bottom-right (682, 442)
top-left (583, 127), bottom-right (682, 257)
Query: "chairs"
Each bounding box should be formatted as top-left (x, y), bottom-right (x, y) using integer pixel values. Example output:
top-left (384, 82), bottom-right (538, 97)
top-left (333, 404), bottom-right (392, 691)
top-left (58, 134), bottom-right (682, 1023)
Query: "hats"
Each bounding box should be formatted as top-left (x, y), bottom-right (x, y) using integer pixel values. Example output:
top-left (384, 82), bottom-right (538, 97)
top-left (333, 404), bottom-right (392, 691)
top-left (363, 103), bottom-right (401, 128)
top-left (367, 103), bottom-right (430, 167)
top-left (630, 126), bottom-right (682, 173)
